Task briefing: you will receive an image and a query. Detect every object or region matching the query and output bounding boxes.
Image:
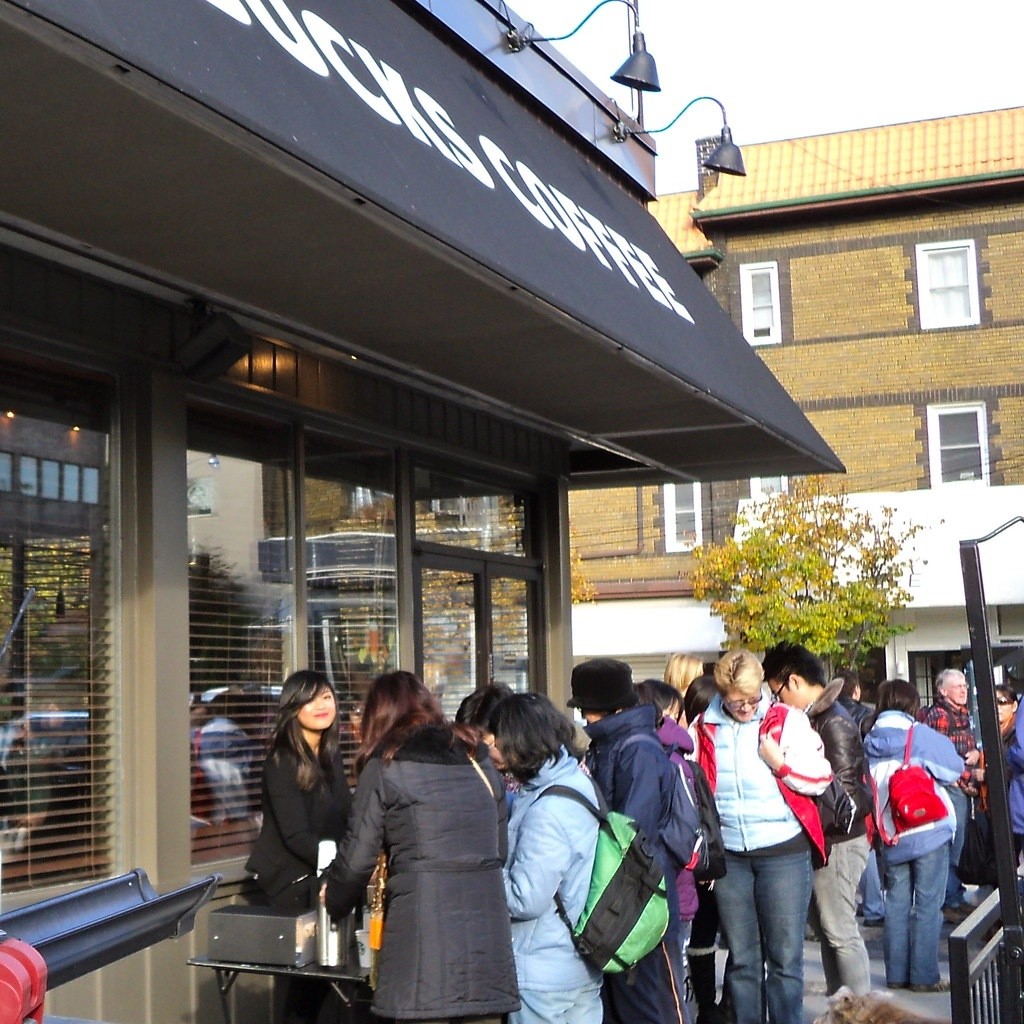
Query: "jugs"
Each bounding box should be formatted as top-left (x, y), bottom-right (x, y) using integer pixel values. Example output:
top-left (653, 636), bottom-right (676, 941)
top-left (317, 878), bottom-right (357, 971)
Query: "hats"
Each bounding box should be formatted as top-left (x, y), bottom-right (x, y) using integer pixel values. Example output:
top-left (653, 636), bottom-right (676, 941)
top-left (566, 657), bottom-right (639, 711)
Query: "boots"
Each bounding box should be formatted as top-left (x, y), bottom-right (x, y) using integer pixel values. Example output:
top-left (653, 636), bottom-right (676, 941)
top-left (681, 942), bottom-right (744, 1023)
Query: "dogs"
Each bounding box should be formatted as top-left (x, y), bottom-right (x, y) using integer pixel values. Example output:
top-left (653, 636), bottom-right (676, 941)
top-left (812, 985), bottom-right (954, 1024)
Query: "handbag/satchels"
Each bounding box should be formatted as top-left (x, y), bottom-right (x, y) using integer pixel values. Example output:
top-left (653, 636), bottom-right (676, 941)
top-left (817, 780), bottom-right (853, 849)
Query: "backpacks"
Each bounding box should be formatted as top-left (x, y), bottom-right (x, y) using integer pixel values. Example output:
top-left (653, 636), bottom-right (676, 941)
top-left (662, 743), bottom-right (728, 883)
top-left (877, 723), bottom-right (949, 847)
top-left (612, 734), bottom-right (706, 872)
top-left (915, 700), bottom-right (959, 741)
top-left (526, 773), bottom-right (670, 987)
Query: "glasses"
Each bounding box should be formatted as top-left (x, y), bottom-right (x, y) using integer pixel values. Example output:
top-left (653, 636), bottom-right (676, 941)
top-left (946, 685), bottom-right (971, 690)
top-left (770, 682), bottom-right (787, 703)
top-left (995, 696), bottom-right (1010, 705)
top-left (721, 690), bottom-right (764, 709)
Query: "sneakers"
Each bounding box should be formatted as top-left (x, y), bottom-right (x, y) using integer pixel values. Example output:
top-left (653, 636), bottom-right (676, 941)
top-left (939, 901), bottom-right (979, 923)
top-left (863, 917), bottom-right (886, 928)
top-left (885, 977), bottom-right (950, 993)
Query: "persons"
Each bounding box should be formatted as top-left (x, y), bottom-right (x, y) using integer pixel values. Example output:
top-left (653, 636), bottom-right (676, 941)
top-left (318, 669), bottom-right (522, 1024)
top-left (680, 648), bottom-right (835, 1024)
top-left (630, 644), bottom-right (1024, 951)
top-left (484, 690), bottom-right (607, 1024)
top-left (242, 668), bottom-right (355, 1024)
top-left (861, 677), bottom-right (968, 993)
top-left (629, 681), bottom-right (701, 1024)
top-left (0, 668), bottom-right (456, 884)
top-left (453, 681), bottom-right (593, 796)
top-left (761, 639), bottom-right (873, 1002)
top-left (564, 654), bottom-right (688, 1024)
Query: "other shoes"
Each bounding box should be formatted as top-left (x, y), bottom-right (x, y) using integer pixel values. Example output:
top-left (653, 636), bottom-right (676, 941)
top-left (851, 903), bottom-right (866, 915)
top-left (805, 929), bottom-right (822, 941)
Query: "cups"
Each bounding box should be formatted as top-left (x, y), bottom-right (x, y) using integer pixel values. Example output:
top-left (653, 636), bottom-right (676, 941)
top-left (354, 926), bottom-right (374, 970)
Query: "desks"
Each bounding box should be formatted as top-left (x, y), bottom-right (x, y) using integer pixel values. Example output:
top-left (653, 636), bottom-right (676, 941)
top-left (186, 954), bottom-right (368, 1024)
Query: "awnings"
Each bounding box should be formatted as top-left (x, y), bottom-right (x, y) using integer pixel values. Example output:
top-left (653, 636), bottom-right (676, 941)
top-left (0, 0), bottom-right (845, 492)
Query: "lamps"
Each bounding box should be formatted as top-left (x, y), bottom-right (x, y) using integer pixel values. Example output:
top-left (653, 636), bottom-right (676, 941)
top-left (176, 296), bottom-right (246, 384)
top-left (614, 97), bottom-right (746, 177)
top-left (507, 0), bottom-right (662, 93)
